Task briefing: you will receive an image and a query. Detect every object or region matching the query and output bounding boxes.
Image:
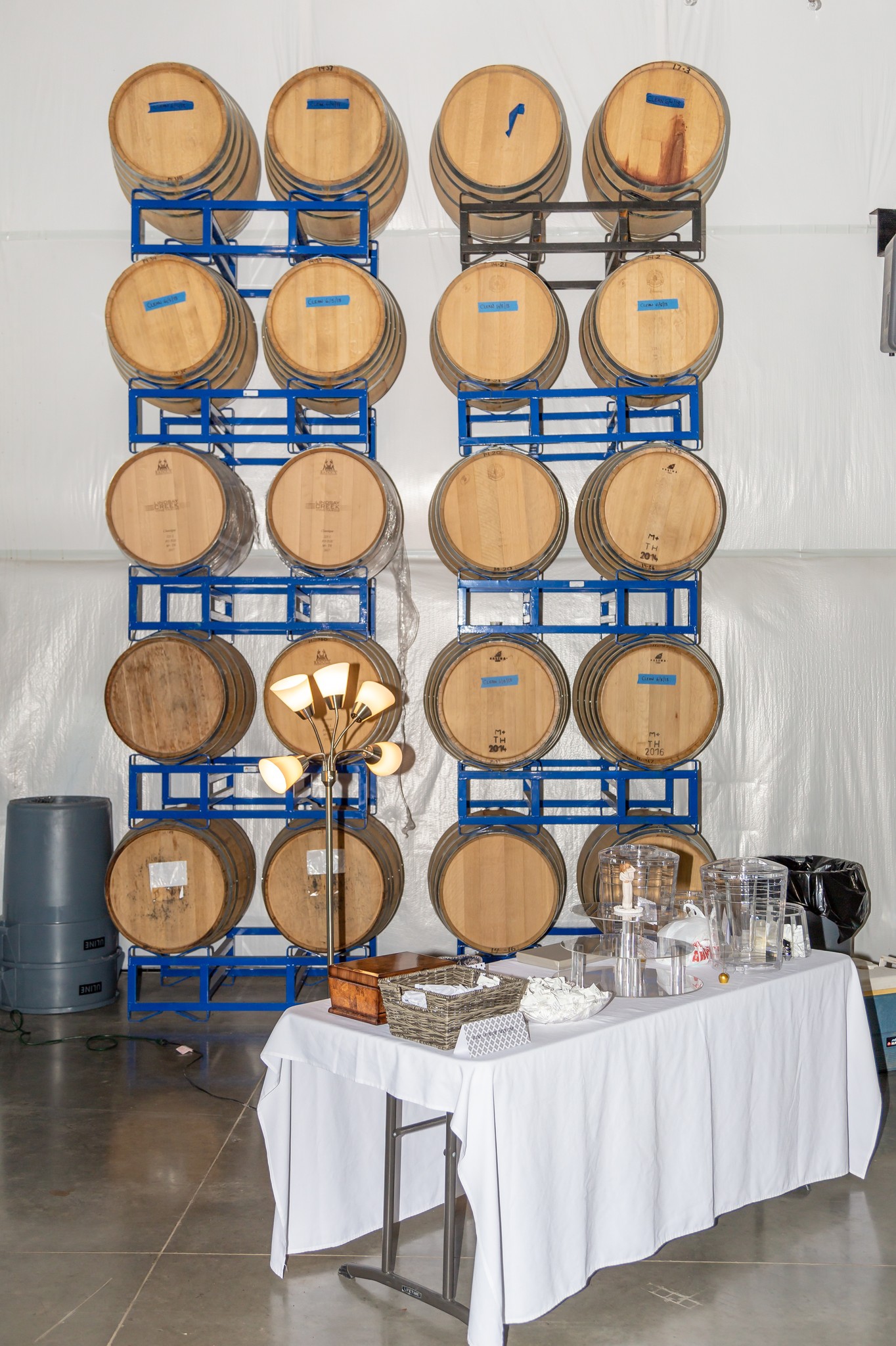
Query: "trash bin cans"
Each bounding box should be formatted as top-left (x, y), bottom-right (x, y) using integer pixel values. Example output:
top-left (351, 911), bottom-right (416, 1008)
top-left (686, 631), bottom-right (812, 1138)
top-left (756, 855), bottom-right (861, 957)
top-left (0, 795), bottom-right (125, 1015)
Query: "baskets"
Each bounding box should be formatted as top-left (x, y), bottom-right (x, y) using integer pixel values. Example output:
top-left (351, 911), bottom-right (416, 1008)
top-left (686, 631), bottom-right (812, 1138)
top-left (379, 965), bottom-right (530, 1049)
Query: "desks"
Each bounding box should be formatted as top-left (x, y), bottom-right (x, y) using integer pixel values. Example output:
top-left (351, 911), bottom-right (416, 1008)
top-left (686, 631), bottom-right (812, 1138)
top-left (257, 927), bottom-right (884, 1346)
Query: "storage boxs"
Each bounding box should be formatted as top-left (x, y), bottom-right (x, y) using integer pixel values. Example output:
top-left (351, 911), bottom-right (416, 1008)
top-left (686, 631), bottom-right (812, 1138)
top-left (851, 957), bottom-right (896, 1072)
top-left (328, 951), bottom-right (457, 1025)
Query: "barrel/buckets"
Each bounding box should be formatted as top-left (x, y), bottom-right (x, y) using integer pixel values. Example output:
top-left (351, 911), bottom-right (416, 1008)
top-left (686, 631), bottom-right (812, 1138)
top-left (102, 806), bottom-right (257, 954)
top-left (264, 65), bottom-right (409, 246)
top-left (598, 844), bottom-right (680, 953)
top-left (0, 796), bottom-right (126, 1016)
top-left (261, 806), bottom-right (405, 954)
top-left (427, 809), bottom-right (567, 957)
top-left (108, 63), bottom-right (261, 245)
top-left (573, 443), bottom-right (724, 581)
top-left (260, 256), bottom-right (407, 417)
top-left (263, 631), bottom-right (404, 766)
top-left (699, 857), bottom-right (788, 975)
top-left (576, 810), bottom-right (716, 935)
top-left (423, 634), bottom-right (572, 773)
top-left (264, 445), bottom-right (405, 580)
top-left (428, 64), bottom-right (572, 243)
top-left (581, 60), bottom-right (731, 241)
top-left (428, 259), bottom-right (570, 415)
top-left (103, 442), bottom-right (257, 578)
top-left (104, 254), bottom-right (260, 415)
top-left (572, 634), bottom-right (725, 770)
top-left (579, 251), bottom-right (724, 409)
top-left (428, 444), bottom-right (569, 582)
top-left (104, 630), bottom-right (258, 765)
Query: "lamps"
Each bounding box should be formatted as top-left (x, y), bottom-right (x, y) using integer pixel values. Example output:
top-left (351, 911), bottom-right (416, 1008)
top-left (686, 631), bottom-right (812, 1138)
top-left (258, 661), bottom-right (402, 998)
